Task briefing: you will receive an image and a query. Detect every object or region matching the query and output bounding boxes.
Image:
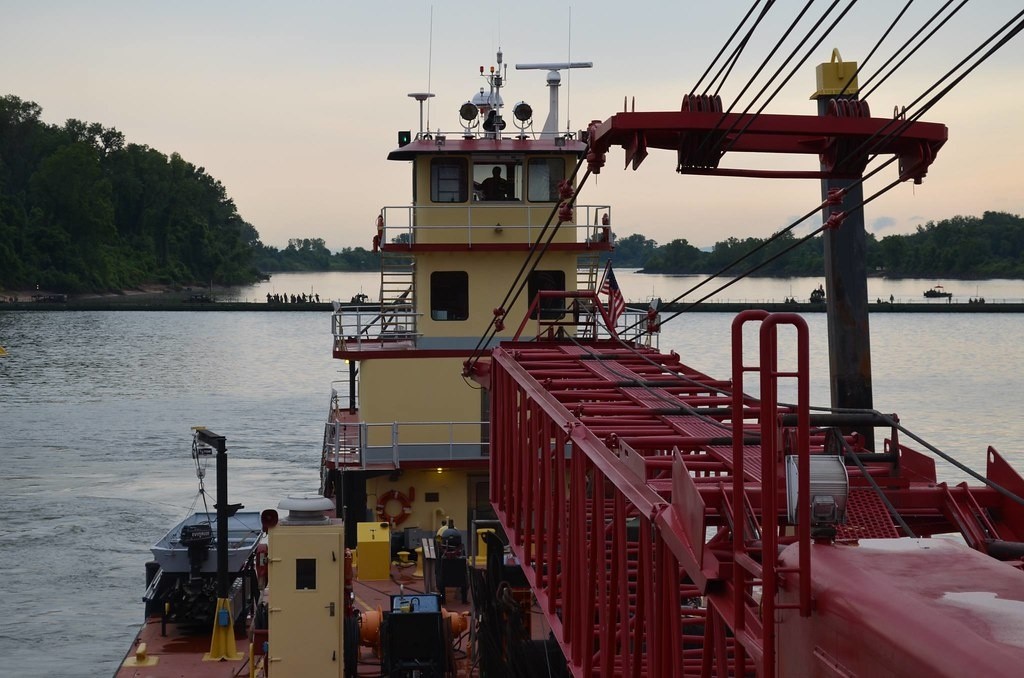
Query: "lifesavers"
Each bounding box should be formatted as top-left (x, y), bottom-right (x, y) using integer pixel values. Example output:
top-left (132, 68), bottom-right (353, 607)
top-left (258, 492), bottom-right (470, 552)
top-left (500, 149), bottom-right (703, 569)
top-left (375, 489), bottom-right (412, 526)
top-left (602, 213), bottom-right (610, 240)
top-left (377, 215), bottom-right (384, 239)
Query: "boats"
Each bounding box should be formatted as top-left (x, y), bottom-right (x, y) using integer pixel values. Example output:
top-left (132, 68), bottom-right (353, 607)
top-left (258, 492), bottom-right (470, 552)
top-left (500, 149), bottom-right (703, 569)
top-left (808, 282), bottom-right (826, 304)
top-left (924, 282), bottom-right (952, 297)
top-left (150, 511), bottom-right (263, 578)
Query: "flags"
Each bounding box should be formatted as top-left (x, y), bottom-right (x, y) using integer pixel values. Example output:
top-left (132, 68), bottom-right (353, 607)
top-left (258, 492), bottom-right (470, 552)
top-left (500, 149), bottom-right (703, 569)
top-left (600, 261), bottom-right (625, 328)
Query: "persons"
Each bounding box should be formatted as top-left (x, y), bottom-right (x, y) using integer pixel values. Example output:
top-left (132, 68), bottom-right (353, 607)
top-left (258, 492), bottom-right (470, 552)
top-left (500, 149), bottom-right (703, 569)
top-left (442, 524), bottom-right (461, 545)
top-left (284, 293), bottom-right (287, 303)
top-left (376, 505), bottom-right (393, 534)
top-left (949, 294), bottom-right (952, 303)
top-left (473, 166), bottom-right (507, 201)
top-left (291, 292), bottom-right (307, 303)
top-left (437, 518), bottom-right (458, 536)
top-left (315, 293), bottom-right (320, 303)
top-left (969, 298), bottom-right (985, 304)
top-left (309, 294), bottom-right (312, 302)
top-left (351, 293), bottom-right (368, 303)
top-left (9, 295), bottom-right (13, 303)
top-left (14, 296), bottom-right (18, 304)
top-left (266, 292), bottom-right (283, 303)
